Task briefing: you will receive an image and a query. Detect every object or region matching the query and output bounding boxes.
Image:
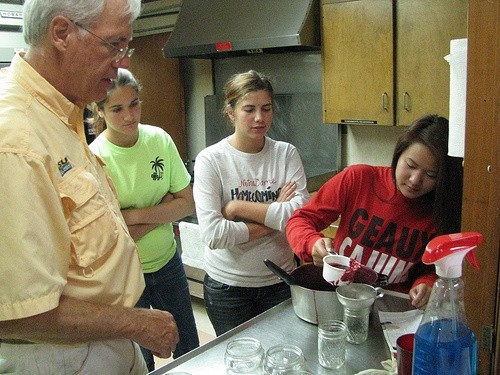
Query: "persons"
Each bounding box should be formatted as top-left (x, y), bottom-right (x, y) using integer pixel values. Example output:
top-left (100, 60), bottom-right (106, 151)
top-left (193, 71), bottom-right (312, 337)
top-left (0, 0), bottom-right (181, 375)
top-left (285, 115), bottom-right (463, 312)
top-left (88, 67), bottom-right (195, 372)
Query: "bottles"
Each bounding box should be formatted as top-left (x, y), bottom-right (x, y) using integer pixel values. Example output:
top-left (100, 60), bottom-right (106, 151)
top-left (316, 319), bottom-right (349, 369)
top-left (262, 344), bottom-right (306, 375)
top-left (224, 337), bottom-right (265, 374)
top-left (342, 306), bottom-right (371, 347)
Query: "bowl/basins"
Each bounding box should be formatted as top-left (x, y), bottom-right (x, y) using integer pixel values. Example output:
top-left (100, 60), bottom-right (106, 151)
top-left (336, 283), bottom-right (386, 309)
top-left (289, 263), bottom-right (388, 325)
top-left (321, 251), bottom-right (358, 286)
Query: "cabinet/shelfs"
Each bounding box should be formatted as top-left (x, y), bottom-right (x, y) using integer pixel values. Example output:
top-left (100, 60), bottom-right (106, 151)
top-left (320, 0), bottom-right (468, 127)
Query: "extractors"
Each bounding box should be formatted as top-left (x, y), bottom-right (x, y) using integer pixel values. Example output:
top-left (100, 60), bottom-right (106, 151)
top-left (159, 0), bottom-right (320, 60)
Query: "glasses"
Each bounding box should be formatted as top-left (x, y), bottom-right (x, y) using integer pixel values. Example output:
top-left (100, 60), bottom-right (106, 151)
top-left (71, 19), bottom-right (135, 63)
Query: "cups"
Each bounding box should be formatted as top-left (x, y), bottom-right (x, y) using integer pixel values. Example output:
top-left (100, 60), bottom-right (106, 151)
top-left (396, 333), bottom-right (417, 375)
